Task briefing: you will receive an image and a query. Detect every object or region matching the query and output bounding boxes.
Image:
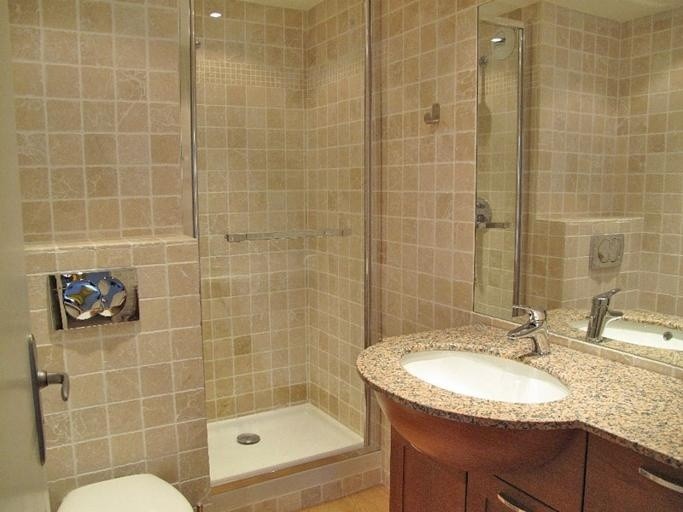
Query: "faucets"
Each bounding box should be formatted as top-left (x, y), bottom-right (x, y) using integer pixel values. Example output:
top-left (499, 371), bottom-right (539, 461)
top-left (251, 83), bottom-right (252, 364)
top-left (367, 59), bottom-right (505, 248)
top-left (584, 287), bottom-right (624, 339)
top-left (506, 303), bottom-right (552, 355)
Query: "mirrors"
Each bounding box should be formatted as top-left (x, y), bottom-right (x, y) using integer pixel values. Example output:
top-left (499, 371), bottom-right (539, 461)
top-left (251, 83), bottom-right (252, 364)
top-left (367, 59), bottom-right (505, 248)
top-left (472, 0), bottom-right (683, 369)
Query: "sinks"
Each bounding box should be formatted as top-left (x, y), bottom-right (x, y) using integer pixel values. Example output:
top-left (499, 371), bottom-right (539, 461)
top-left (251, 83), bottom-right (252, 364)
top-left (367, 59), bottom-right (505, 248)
top-left (568, 314), bottom-right (683, 353)
top-left (399, 343), bottom-right (570, 403)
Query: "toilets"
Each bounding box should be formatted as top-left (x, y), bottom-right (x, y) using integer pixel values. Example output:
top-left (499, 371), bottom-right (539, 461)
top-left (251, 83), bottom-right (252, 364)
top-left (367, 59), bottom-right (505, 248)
top-left (55, 473), bottom-right (194, 512)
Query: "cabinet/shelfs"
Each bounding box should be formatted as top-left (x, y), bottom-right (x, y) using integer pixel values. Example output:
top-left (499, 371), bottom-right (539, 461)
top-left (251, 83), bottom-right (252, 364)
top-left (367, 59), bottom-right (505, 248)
top-left (372, 375), bottom-right (683, 512)
